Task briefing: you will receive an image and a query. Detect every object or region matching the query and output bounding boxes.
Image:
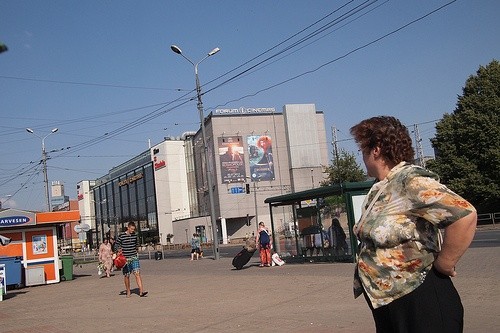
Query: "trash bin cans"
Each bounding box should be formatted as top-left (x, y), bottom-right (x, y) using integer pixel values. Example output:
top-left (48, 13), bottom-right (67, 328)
top-left (61, 255), bottom-right (75, 280)
top-left (0, 256), bottom-right (23, 289)
top-left (285, 235), bottom-right (292, 249)
top-left (155, 251), bottom-right (162, 260)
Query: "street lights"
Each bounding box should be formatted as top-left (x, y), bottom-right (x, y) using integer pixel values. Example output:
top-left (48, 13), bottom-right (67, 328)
top-left (246, 175), bottom-right (263, 231)
top-left (311, 169), bottom-right (315, 189)
top-left (91, 199), bottom-right (106, 244)
top-left (25, 127), bottom-right (59, 211)
top-left (170, 44), bottom-right (221, 260)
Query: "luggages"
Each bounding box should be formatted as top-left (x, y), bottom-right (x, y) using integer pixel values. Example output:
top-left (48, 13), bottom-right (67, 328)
top-left (231, 247), bottom-right (257, 270)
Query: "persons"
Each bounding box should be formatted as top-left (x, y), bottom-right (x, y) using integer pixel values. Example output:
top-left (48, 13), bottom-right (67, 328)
top-left (246, 231), bottom-right (254, 240)
top-left (257, 222), bottom-right (271, 267)
top-left (349, 116), bottom-right (478, 333)
top-left (327, 218), bottom-right (348, 255)
top-left (113, 222), bottom-right (148, 297)
top-left (324, 238), bottom-right (329, 248)
top-left (98, 236), bottom-right (121, 278)
top-left (221, 146), bottom-right (242, 161)
top-left (189, 233), bottom-right (201, 260)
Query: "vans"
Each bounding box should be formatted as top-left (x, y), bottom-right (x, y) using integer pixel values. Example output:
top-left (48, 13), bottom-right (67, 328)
top-left (284, 221), bottom-right (300, 239)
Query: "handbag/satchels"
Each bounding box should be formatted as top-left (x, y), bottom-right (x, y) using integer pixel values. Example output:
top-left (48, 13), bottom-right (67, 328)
top-left (272, 252), bottom-right (285, 266)
top-left (96, 262), bottom-right (105, 276)
top-left (114, 254), bottom-right (127, 270)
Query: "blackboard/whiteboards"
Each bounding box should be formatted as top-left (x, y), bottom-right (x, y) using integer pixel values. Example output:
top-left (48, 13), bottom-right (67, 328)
top-left (0, 264), bottom-right (6, 295)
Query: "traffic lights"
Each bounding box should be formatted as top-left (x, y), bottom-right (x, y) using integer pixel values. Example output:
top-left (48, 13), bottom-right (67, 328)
top-left (246, 184), bottom-right (250, 194)
top-left (249, 219), bottom-right (252, 226)
top-left (246, 219), bottom-right (248, 226)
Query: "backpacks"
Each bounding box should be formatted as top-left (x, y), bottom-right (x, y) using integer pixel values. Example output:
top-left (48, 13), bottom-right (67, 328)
top-left (258, 229), bottom-right (271, 248)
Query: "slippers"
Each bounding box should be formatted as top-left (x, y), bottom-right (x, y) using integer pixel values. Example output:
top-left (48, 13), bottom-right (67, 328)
top-left (140, 290), bottom-right (148, 297)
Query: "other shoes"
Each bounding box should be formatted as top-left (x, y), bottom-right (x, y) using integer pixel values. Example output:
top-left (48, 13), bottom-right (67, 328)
top-left (259, 264), bottom-right (265, 267)
top-left (107, 273), bottom-right (110, 277)
top-left (265, 264), bottom-right (270, 267)
